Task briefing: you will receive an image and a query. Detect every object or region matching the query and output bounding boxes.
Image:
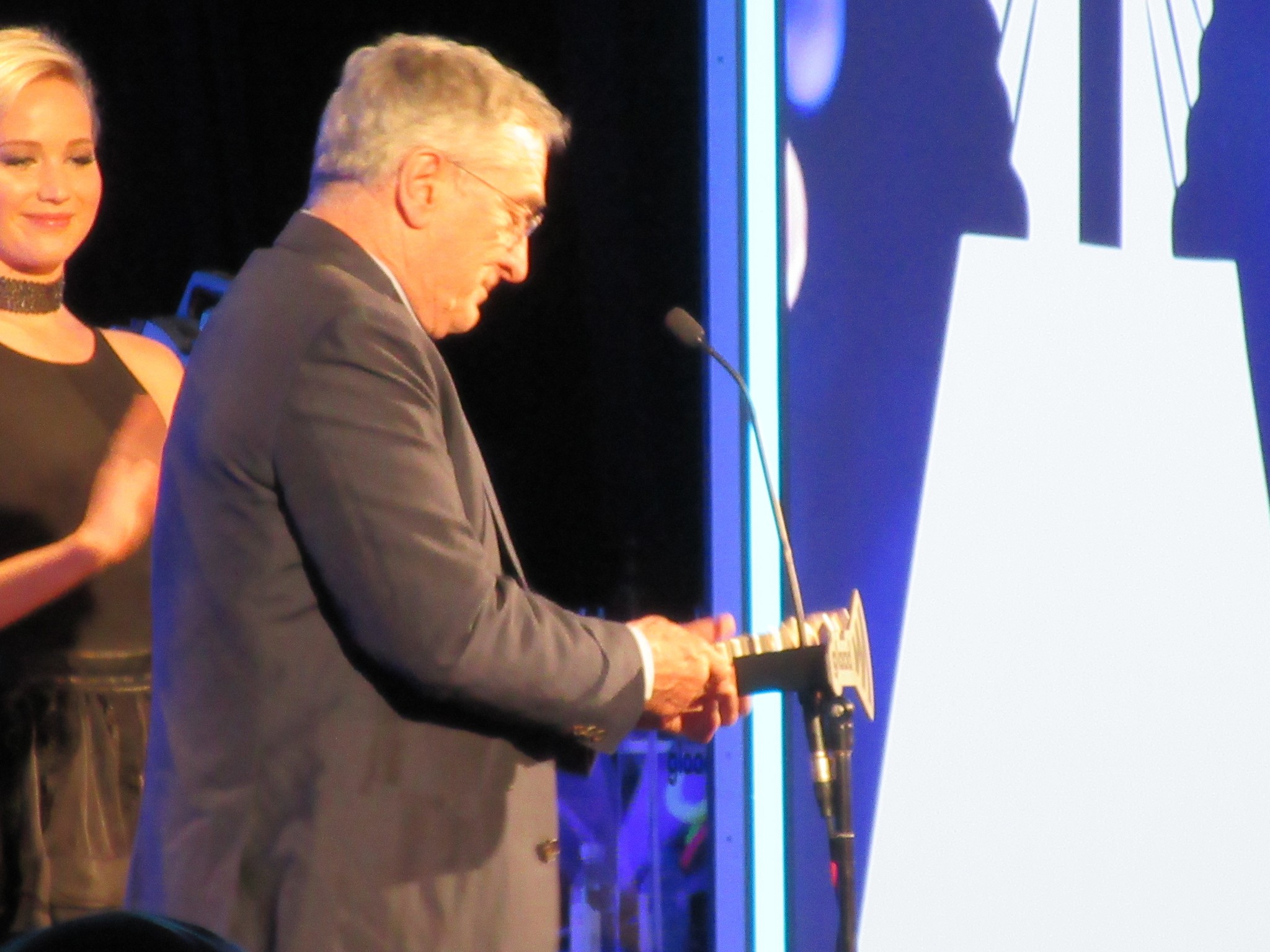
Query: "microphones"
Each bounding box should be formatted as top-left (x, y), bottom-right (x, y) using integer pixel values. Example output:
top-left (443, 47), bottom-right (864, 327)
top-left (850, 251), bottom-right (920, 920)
top-left (662, 303), bottom-right (810, 649)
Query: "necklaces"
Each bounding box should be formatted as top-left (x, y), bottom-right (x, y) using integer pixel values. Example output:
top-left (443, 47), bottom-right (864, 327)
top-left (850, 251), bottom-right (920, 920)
top-left (0, 275), bottom-right (65, 315)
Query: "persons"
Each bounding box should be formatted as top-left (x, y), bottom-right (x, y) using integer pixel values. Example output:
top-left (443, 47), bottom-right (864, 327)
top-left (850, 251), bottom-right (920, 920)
top-left (124, 31), bottom-right (748, 952)
top-left (0, 22), bottom-right (194, 952)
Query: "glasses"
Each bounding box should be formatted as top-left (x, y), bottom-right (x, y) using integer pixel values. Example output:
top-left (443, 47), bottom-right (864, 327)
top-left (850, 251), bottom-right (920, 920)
top-left (445, 158), bottom-right (544, 239)
